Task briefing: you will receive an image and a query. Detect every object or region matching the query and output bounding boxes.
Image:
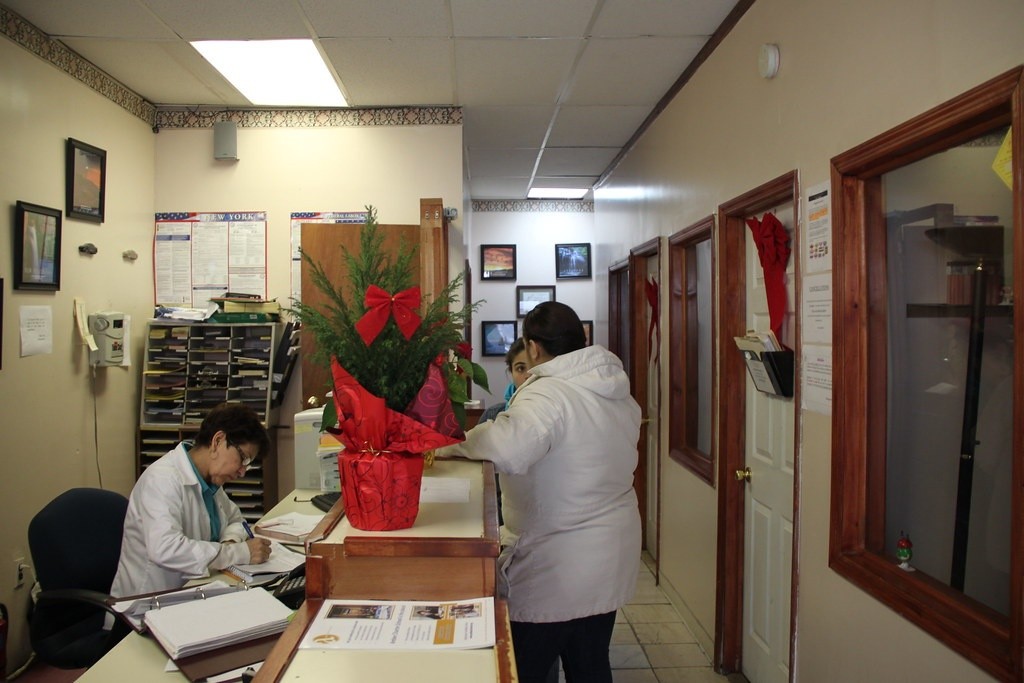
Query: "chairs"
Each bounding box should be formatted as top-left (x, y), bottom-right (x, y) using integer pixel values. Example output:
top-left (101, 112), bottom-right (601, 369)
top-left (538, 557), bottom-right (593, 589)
top-left (27, 487), bottom-right (129, 670)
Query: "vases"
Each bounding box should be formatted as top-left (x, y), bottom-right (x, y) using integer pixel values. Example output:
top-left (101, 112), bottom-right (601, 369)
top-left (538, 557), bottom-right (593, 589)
top-left (422, 447), bottom-right (436, 470)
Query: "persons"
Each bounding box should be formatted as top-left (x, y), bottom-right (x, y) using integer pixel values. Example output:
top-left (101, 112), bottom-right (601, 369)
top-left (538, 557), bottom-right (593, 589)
top-left (417, 608), bottom-right (444, 619)
top-left (432, 301), bottom-right (643, 683)
top-left (110, 401), bottom-right (272, 630)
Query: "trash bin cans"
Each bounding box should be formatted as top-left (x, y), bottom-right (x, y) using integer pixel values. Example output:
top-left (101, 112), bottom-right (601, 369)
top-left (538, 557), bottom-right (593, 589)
top-left (464, 398), bottom-right (484, 409)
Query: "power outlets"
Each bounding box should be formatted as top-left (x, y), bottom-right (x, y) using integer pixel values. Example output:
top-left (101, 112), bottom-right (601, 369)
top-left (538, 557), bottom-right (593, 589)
top-left (13, 557), bottom-right (26, 589)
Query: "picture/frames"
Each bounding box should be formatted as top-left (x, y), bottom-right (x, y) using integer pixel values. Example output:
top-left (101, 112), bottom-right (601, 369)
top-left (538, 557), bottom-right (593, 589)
top-left (481, 244), bottom-right (517, 282)
top-left (481, 321), bottom-right (518, 357)
top-left (516, 285), bottom-right (556, 319)
top-left (65, 137), bottom-right (107, 223)
top-left (13, 200), bottom-right (62, 292)
top-left (581, 320), bottom-right (594, 347)
top-left (553, 243), bottom-right (593, 279)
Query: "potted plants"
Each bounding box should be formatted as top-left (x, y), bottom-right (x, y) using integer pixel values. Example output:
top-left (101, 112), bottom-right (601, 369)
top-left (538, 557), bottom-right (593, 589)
top-left (275, 204), bottom-right (487, 533)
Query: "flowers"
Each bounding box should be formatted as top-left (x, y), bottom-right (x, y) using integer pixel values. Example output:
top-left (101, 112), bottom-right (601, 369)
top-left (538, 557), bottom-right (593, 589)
top-left (421, 313), bottom-right (493, 427)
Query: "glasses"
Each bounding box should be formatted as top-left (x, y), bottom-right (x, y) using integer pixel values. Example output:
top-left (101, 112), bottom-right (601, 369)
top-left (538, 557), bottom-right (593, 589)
top-left (229, 438), bottom-right (251, 472)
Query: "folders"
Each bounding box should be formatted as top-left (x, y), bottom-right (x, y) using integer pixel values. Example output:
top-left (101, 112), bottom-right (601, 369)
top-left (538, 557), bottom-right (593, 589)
top-left (106, 580), bottom-right (293, 683)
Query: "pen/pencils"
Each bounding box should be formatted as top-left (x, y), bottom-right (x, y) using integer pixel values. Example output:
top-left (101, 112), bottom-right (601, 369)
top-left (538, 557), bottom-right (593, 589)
top-left (241, 520), bottom-right (254, 538)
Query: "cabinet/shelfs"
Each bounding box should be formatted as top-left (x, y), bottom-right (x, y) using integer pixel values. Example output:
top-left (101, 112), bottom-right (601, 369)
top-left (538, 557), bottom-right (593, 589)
top-left (136, 318), bottom-right (287, 528)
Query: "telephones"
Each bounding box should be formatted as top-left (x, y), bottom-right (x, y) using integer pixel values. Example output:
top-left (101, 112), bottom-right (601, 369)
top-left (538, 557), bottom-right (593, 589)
top-left (273, 562), bottom-right (307, 608)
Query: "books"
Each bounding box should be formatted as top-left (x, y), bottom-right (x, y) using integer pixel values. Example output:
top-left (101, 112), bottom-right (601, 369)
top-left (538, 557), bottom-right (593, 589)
top-left (735, 329), bottom-right (783, 358)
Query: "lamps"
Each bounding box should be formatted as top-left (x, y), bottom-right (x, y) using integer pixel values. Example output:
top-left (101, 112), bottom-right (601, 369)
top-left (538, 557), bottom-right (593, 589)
top-left (925, 224), bottom-right (1011, 592)
